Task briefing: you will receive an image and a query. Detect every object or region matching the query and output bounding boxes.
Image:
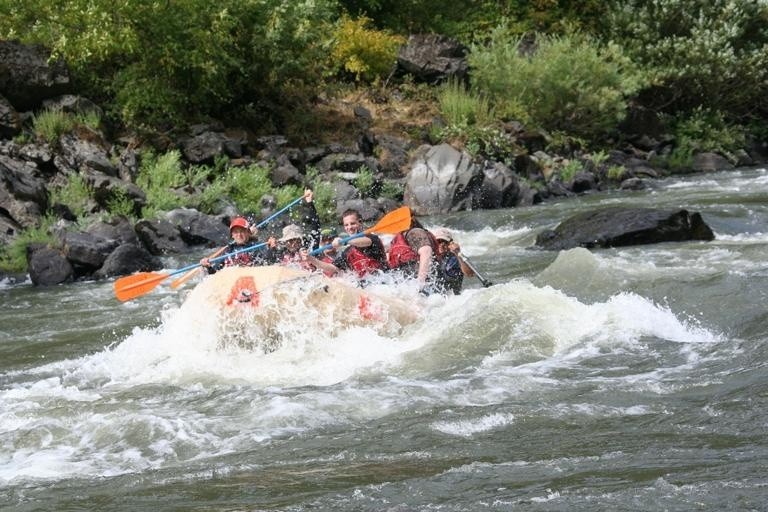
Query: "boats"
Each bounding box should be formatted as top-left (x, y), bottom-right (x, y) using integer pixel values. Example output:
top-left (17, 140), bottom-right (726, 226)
top-left (177, 260), bottom-right (431, 348)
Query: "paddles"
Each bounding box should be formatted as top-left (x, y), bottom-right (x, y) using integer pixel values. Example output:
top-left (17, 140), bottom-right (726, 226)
top-left (114, 238), bottom-right (276, 302)
top-left (172, 192), bottom-right (312, 287)
top-left (305, 207), bottom-right (411, 256)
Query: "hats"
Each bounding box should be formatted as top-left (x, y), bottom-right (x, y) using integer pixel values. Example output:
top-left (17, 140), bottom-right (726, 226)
top-left (433, 228), bottom-right (454, 244)
top-left (228, 216), bottom-right (251, 231)
top-left (320, 227), bottom-right (339, 241)
top-left (279, 223), bottom-right (307, 241)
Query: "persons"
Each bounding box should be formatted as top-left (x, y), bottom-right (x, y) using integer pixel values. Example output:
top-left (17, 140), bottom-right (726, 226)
top-left (201, 188), bottom-right (473, 302)
